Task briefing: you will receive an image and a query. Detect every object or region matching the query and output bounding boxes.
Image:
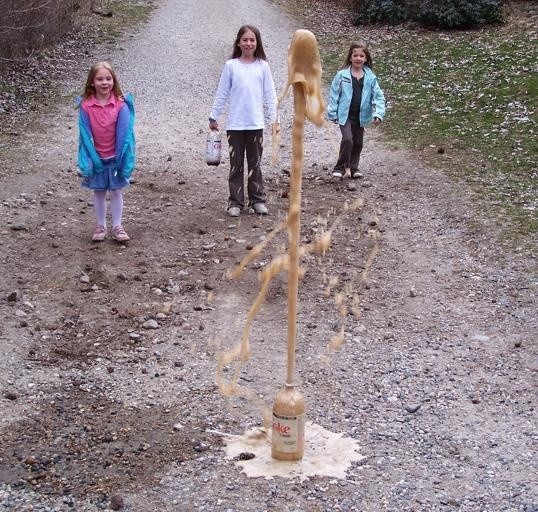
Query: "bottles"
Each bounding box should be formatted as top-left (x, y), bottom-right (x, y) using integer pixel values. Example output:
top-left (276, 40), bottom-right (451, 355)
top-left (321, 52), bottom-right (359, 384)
top-left (206, 129), bottom-right (222, 166)
top-left (272, 384), bottom-right (305, 460)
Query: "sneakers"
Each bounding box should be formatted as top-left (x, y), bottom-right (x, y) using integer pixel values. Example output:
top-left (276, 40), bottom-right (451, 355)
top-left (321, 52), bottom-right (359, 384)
top-left (227, 206), bottom-right (241, 217)
top-left (91, 224), bottom-right (107, 242)
top-left (248, 202), bottom-right (269, 215)
top-left (332, 169), bottom-right (364, 178)
top-left (110, 225), bottom-right (130, 242)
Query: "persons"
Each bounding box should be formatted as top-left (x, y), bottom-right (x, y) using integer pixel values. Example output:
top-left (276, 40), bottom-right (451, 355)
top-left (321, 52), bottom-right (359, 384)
top-left (325, 44), bottom-right (385, 182)
top-left (73, 62), bottom-right (137, 244)
top-left (209, 23), bottom-right (280, 216)
